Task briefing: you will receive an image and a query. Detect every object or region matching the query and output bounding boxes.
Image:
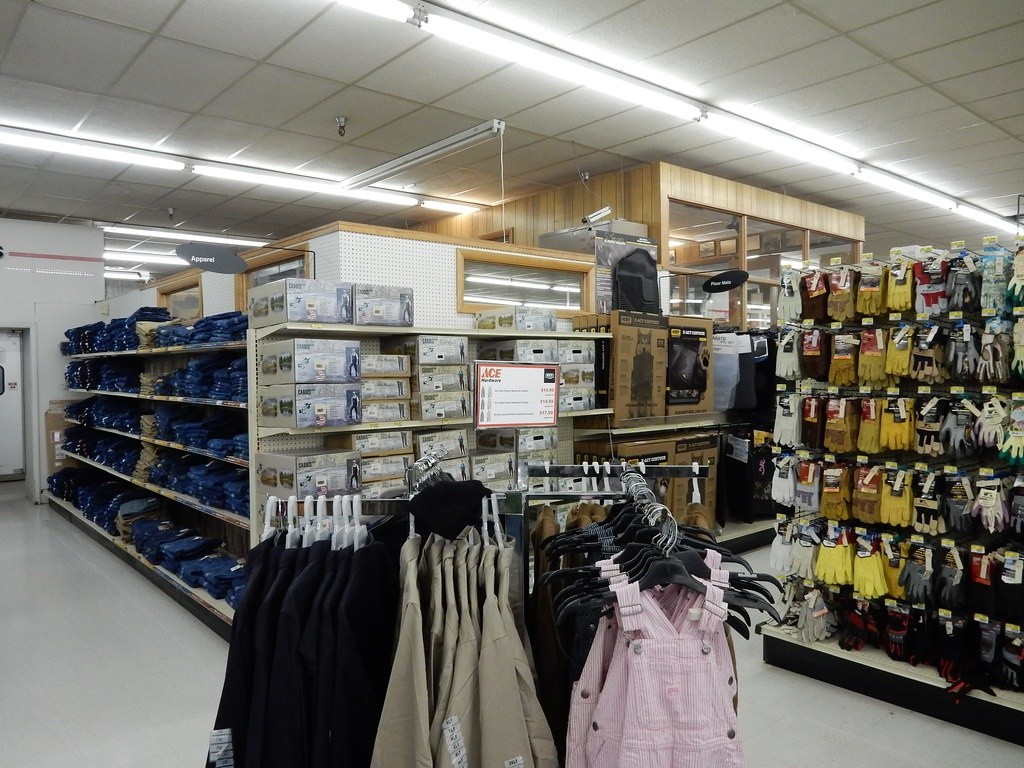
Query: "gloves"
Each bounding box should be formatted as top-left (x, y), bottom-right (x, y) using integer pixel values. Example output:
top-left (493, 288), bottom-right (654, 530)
top-left (769, 250), bottom-right (1024, 691)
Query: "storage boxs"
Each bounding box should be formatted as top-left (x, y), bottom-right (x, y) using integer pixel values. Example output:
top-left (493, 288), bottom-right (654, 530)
top-left (45, 401), bottom-right (80, 476)
top-left (246, 277), bottom-right (717, 528)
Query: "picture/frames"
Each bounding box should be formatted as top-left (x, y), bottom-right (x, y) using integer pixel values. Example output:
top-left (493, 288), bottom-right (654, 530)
top-left (747, 234), bottom-right (761, 251)
top-left (783, 229), bottom-right (805, 248)
top-left (670, 249), bottom-right (676, 265)
top-left (698, 241), bottom-right (716, 258)
top-left (719, 239), bottom-right (737, 255)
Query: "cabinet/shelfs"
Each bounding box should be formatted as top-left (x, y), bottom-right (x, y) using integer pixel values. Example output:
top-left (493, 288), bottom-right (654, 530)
top-left (573, 420), bottom-right (782, 558)
top-left (42, 321), bottom-right (614, 646)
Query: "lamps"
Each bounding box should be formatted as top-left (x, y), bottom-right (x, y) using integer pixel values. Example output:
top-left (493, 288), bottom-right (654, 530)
top-left (326, 0), bottom-right (1024, 234)
top-left (0, 125), bottom-right (491, 214)
top-left (342, 118), bottom-right (506, 190)
top-left (93, 221), bottom-right (276, 247)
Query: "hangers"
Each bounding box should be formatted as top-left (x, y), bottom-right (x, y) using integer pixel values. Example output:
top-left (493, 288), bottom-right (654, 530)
top-left (541, 461), bottom-right (785, 641)
top-left (260, 493), bottom-right (507, 553)
